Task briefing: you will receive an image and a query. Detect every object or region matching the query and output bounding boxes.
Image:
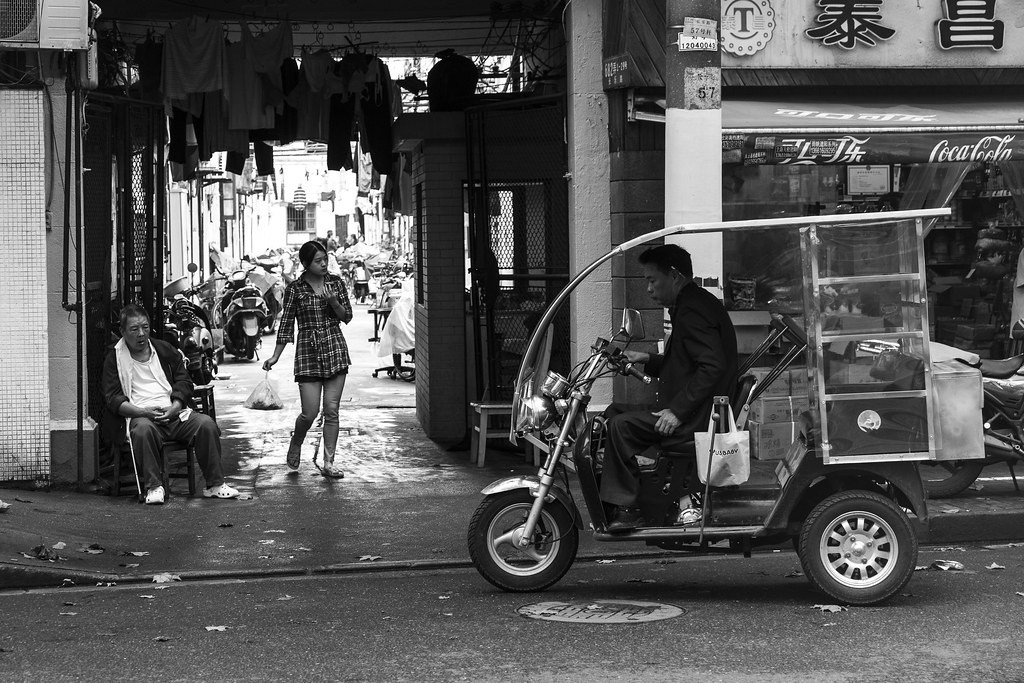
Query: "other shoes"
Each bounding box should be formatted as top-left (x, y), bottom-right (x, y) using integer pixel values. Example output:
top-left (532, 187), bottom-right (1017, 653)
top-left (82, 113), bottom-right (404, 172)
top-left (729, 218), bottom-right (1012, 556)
top-left (321, 466), bottom-right (344, 478)
top-left (608, 506), bottom-right (655, 531)
top-left (287, 437), bottom-right (301, 470)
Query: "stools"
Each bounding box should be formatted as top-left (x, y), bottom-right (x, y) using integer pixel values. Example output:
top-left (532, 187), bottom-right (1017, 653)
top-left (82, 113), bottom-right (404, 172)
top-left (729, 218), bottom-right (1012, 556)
top-left (111, 385), bottom-right (216, 502)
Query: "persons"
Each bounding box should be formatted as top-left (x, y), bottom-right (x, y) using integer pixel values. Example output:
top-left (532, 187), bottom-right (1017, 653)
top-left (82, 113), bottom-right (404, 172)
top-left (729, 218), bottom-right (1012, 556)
top-left (325, 230), bottom-right (364, 252)
top-left (598, 243), bottom-right (738, 535)
top-left (102, 304), bottom-right (239, 503)
top-left (263, 240), bottom-right (353, 477)
top-left (354, 261), bottom-right (368, 303)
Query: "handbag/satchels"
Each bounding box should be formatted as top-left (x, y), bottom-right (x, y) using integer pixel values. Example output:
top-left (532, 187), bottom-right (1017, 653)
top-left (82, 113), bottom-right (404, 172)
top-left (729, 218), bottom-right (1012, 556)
top-left (694, 404), bottom-right (750, 487)
top-left (243, 371), bottom-right (284, 410)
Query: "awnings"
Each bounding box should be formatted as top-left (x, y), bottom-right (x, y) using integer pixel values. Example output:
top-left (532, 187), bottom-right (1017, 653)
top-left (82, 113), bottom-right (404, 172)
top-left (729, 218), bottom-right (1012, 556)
top-left (652, 97), bottom-right (1024, 162)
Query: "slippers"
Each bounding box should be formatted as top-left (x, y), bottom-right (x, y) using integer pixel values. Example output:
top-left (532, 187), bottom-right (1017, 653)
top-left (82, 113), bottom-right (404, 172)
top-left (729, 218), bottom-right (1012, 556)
top-left (146, 486), bottom-right (165, 504)
top-left (202, 482), bottom-right (240, 498)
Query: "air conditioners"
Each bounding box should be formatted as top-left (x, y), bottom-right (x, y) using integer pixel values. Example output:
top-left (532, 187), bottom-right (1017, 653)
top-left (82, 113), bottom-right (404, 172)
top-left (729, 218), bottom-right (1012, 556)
top-left (0, 0), bottom-right (90, 51)
top-left (198, 151), bottom-right (224, 174)
top-left (204, 171), bottom-right (233, 183)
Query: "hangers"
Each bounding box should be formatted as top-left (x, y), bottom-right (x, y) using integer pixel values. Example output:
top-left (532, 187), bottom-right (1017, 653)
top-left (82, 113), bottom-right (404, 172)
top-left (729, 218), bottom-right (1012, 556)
top-left (133, 18), bottom-right (380, 59)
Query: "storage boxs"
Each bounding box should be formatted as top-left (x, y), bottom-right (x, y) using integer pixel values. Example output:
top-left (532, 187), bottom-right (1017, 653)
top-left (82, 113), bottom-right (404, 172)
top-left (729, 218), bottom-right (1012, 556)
top-left (748, 397), bottom-right (818, 423)
top-left (931, 360), bottom-right (985, 462)
top-left (746, 420), bottom-right (807, 462)
top-left (750, 368), bottom-right (815, 397)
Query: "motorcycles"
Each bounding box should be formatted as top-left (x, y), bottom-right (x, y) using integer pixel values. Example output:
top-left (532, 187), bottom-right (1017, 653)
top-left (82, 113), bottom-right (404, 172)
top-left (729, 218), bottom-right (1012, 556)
top-left (207, 238), bottom-right (414, 362)
top-left (466, 207), bottom-right (984, 607)
top-left (161, 264), bottom-right (226, 385)
top-left (843, 319), bottom-right (1024, 498)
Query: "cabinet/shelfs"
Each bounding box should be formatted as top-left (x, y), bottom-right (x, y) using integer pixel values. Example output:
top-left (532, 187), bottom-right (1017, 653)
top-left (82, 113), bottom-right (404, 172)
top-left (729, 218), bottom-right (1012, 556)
top-left (722, 161), bottom-right (1024, 356)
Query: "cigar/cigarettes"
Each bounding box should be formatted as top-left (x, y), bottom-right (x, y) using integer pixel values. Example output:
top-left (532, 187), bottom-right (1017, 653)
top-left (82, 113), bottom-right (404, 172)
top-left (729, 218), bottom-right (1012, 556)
top-left (671, 265), bottom-right (685, 279)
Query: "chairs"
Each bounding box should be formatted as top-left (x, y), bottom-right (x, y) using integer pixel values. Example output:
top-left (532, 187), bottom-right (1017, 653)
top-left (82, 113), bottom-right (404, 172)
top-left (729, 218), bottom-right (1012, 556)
top-left (470, 324), bottom-right (554, 470)
